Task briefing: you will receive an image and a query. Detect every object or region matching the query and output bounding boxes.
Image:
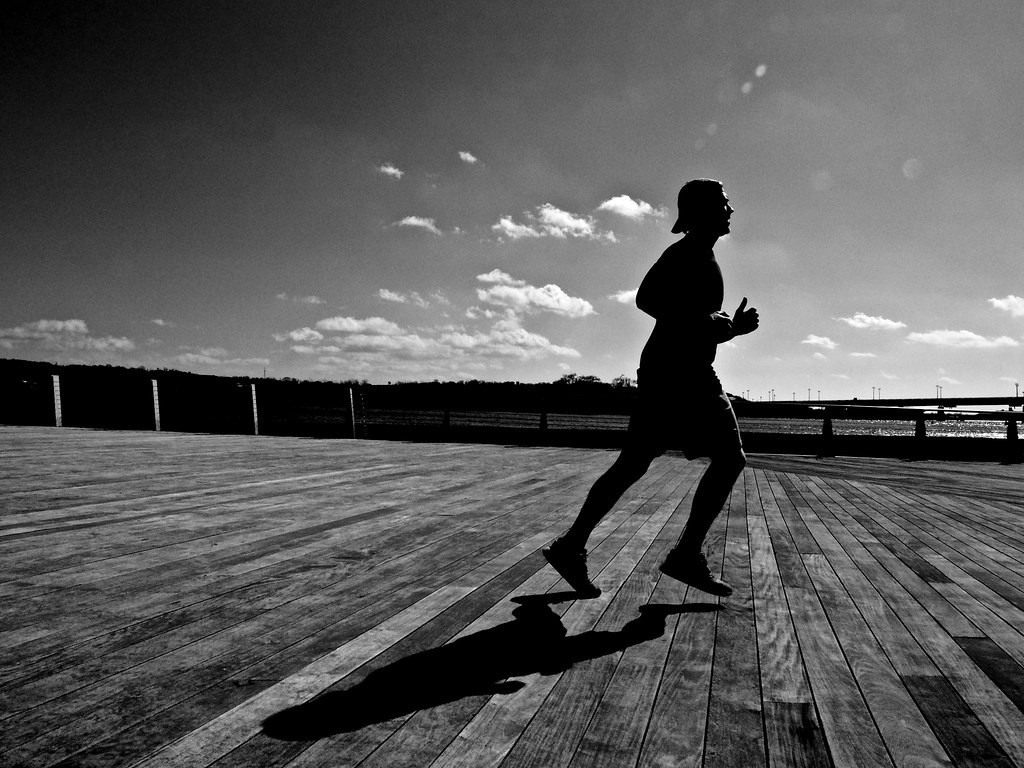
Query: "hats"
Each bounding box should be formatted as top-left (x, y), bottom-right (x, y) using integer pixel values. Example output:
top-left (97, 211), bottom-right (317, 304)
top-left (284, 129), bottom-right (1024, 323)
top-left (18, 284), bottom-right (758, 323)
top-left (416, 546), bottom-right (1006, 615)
top-left (671, 178), bottom-right (725, 233)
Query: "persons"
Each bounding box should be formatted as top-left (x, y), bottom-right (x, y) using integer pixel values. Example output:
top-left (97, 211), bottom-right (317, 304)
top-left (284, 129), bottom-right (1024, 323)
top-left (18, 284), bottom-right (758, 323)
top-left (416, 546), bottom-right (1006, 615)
top-left (542, 178), bottom-right (759, 597)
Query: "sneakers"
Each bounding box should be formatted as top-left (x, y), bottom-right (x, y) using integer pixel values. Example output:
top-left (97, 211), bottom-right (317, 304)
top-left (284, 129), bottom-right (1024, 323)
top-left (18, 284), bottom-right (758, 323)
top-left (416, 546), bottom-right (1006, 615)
top-left (658, 549), bottom-right (733, 597)
top-left (541, 538), bottom-right (601, 598)
